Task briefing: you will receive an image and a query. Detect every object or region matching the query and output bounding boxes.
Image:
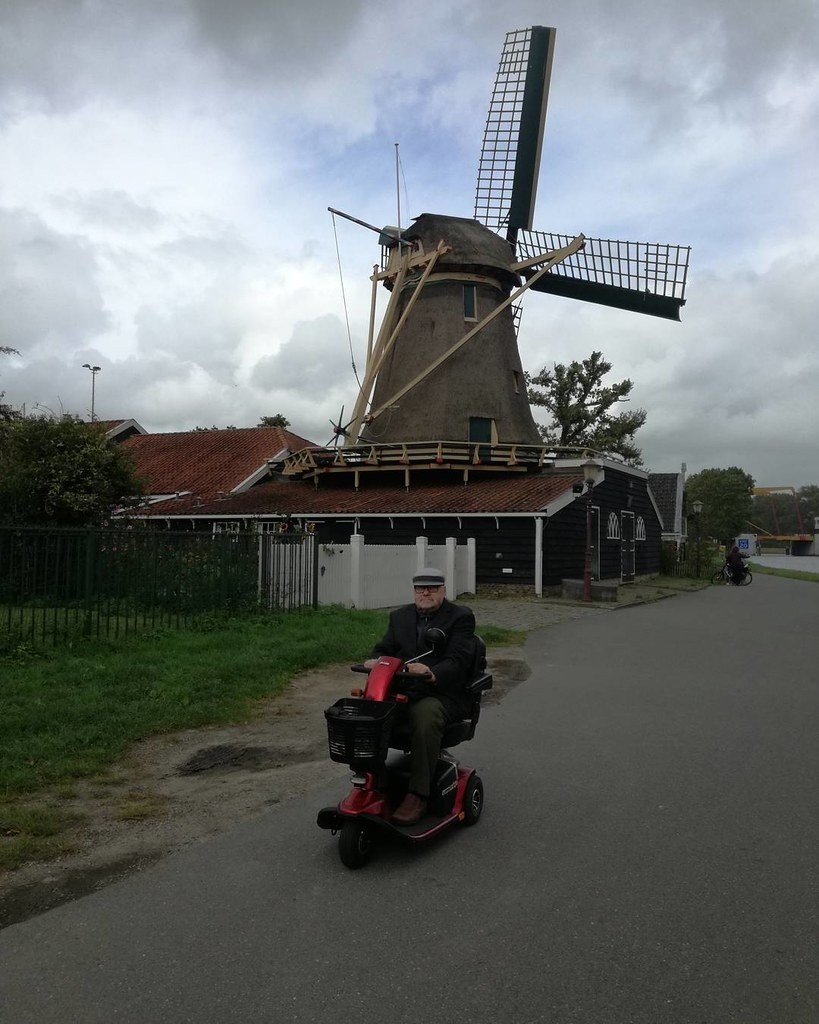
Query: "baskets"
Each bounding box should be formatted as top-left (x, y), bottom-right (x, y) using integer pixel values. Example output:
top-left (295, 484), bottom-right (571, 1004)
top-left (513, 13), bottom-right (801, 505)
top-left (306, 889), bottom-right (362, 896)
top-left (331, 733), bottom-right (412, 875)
top-left (324, 698), bottom-right (397, 765)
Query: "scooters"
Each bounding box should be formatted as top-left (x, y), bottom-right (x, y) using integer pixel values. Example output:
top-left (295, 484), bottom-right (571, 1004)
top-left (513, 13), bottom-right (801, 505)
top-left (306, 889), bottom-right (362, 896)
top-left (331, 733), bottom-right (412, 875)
top-left (316, 657), bottom-right (493, 870)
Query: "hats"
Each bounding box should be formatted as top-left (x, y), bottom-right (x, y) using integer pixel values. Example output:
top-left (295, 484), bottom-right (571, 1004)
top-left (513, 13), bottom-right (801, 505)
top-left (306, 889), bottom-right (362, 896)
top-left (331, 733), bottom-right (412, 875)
top-left (413, 568), bottom-right (445, 585)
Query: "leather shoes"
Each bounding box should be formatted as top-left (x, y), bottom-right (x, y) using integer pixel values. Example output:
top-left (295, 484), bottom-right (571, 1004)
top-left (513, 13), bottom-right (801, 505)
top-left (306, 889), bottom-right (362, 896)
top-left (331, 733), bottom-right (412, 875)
top-left (391, 793), bottom-right (428, 824)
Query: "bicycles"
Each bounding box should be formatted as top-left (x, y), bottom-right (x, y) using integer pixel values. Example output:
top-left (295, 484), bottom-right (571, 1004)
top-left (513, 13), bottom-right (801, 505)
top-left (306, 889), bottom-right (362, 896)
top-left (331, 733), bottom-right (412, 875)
top-left (711, 560), bottom-right (752, 586)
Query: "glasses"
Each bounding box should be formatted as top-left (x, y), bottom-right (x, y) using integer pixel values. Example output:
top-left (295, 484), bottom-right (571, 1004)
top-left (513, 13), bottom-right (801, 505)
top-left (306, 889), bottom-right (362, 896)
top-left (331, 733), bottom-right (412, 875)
top-left (414, 585), bottom-right (443, 593)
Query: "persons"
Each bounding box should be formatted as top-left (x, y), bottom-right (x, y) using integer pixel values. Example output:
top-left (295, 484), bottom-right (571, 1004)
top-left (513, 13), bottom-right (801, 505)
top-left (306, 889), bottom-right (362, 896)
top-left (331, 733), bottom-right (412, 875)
top-left (364, 566), bottom-right (475, 822)
top-left (726, 546), bottom-right (749, 585)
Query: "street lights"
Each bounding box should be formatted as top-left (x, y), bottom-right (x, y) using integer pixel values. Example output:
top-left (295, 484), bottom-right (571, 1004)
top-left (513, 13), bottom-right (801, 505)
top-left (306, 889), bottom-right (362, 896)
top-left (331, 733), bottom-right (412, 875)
top-left (83, 364), bottom-right (101, 423)
top-left (579, 459), bottom-right (601, 603)
top-left (692, 500), bottom-right (704, 578)
top-left (395, 143), bottom-right (403, 257)
